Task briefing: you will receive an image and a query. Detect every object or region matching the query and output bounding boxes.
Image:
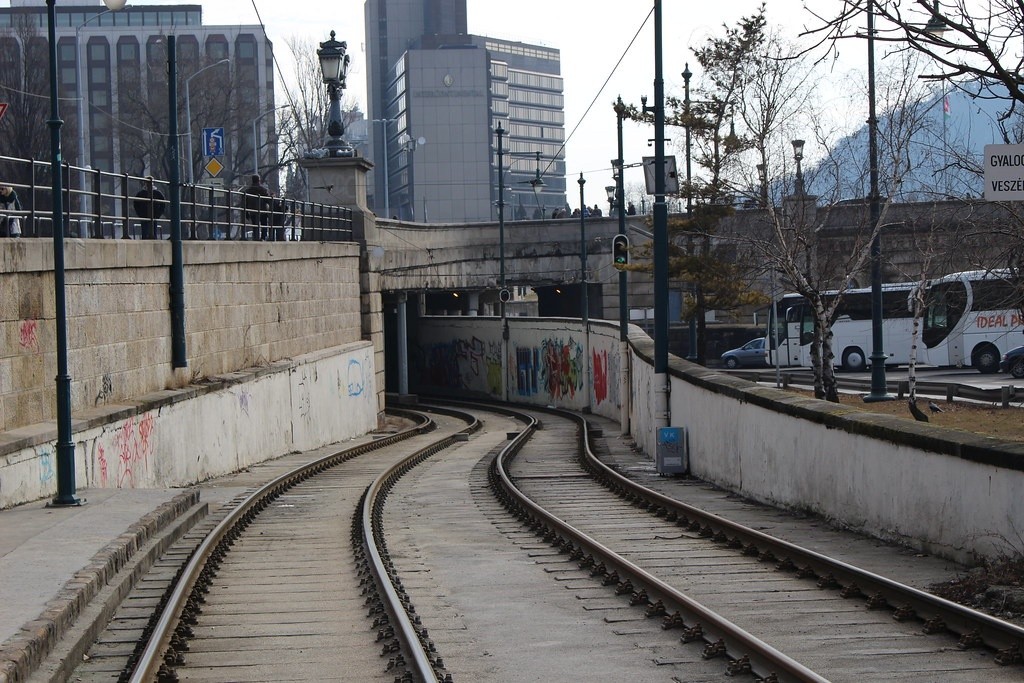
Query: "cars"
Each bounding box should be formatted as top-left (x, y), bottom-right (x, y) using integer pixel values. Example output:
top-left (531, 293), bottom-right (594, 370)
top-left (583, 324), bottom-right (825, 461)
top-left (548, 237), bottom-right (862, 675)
top-left (999, 346), bottom-right (1024, 379)
top-left (721, 337), bottom-right (766, 369)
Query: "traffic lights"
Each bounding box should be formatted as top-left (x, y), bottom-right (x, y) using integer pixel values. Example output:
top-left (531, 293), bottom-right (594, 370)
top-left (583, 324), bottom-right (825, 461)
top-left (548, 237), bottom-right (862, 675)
top-left (612, 235), bottom-right (630, 272)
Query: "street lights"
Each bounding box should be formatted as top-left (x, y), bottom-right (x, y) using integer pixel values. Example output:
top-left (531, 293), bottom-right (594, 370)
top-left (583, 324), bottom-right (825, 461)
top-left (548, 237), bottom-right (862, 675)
top-left (75, 1), bottom-right (134, 241)
top-left (372, 118), bottom-right (398, 218)
top-left (46, 0), bottom-right (126, 506)
top-left (252, 103), bottom-right (291, 241)
top-left (184, 58), bottom-right (231, 246)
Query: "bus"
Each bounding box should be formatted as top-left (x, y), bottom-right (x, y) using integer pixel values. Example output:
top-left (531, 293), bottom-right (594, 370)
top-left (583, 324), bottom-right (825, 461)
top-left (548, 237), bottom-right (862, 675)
top-left (764, 278), bottom-right (951, 373)
top-left (908, 267), bottom-right (1024, 375)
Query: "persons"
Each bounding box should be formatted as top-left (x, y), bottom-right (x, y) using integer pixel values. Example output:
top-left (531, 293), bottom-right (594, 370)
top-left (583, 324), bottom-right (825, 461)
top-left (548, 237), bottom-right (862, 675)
top-left (133, 176), bottom-right (166, 241)
top-left (244, 174), bottom-right (287, 242)
top-left (552, 202), bottom-right (636, 220)
top-left (0, 184), bottom-right (22, 238)
top-left (966, 192), bottom-right (985, 199)
